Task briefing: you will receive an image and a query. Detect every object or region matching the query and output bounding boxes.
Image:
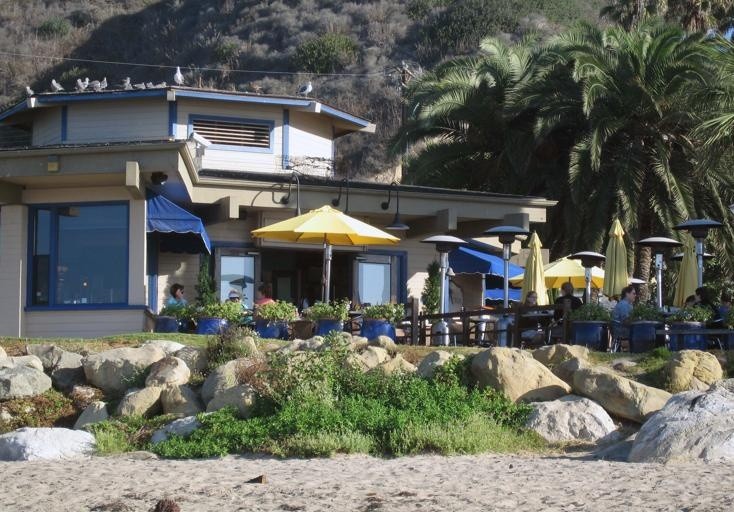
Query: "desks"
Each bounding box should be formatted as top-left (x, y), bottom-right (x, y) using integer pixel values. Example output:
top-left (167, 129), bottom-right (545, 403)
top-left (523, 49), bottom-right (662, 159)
top-left (522, 313), bottom-right (554, 345)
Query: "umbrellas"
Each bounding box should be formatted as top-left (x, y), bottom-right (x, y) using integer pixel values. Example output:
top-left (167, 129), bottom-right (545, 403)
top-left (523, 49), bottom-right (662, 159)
top-left (251, 205), bottom-right (400, 303)
top-left (521, 231), bottom-right (550, 306)
top-left (509, 254), bottom-right (605, 290)
top-left (602, 218), bottom-right (628, 297)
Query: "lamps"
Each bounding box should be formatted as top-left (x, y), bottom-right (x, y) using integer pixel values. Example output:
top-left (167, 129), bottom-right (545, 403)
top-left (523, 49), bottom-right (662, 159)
top-left (331, 177), bottom-right (350, 215)
top-left (280, 172), bottom-right (302, 217)
top-left (380, 181), bottom-right (411, 232)
top-left (150, 171), bottom-right (170, 185)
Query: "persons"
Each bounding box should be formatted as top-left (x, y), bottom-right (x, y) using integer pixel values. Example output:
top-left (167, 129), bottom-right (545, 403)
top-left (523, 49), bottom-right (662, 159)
top-left (683, 287), bottom-right (723, 346)
top-left (544, 282), bottom-right (583, 345)
top-left (256, 282), bottom-right (276, 306)
top-left (229, 289), bottom-right (252, 325)
top-left (612, 286), bottom-right (637, 338)
top-left (519, 291), bottom-right (545, 346)
top-left (167, 284), bottom-right (188, 308)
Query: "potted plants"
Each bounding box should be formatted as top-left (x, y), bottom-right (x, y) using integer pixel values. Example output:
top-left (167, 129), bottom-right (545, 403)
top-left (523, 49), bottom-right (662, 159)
top-left (153, 296), bottom-right (406, 345)
top-left (570, 299), bottom-right (713, 352)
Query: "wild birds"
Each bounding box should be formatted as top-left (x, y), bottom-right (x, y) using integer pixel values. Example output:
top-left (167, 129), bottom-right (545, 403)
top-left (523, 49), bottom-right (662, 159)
top-left (249, 81), bottom-right (262, 93)
top-left (174, 66), bottom-right (191, 87)
top-left (297, 80), bottom-right (313, 97)
top-left (123, 77), bottom-right (167, 90)
top-left (25, 85), bottom-right (34, 95)
top-left (51, 78), bottom-right (65, 92)
top-left (75, 76), bottom-right (108, 92)
